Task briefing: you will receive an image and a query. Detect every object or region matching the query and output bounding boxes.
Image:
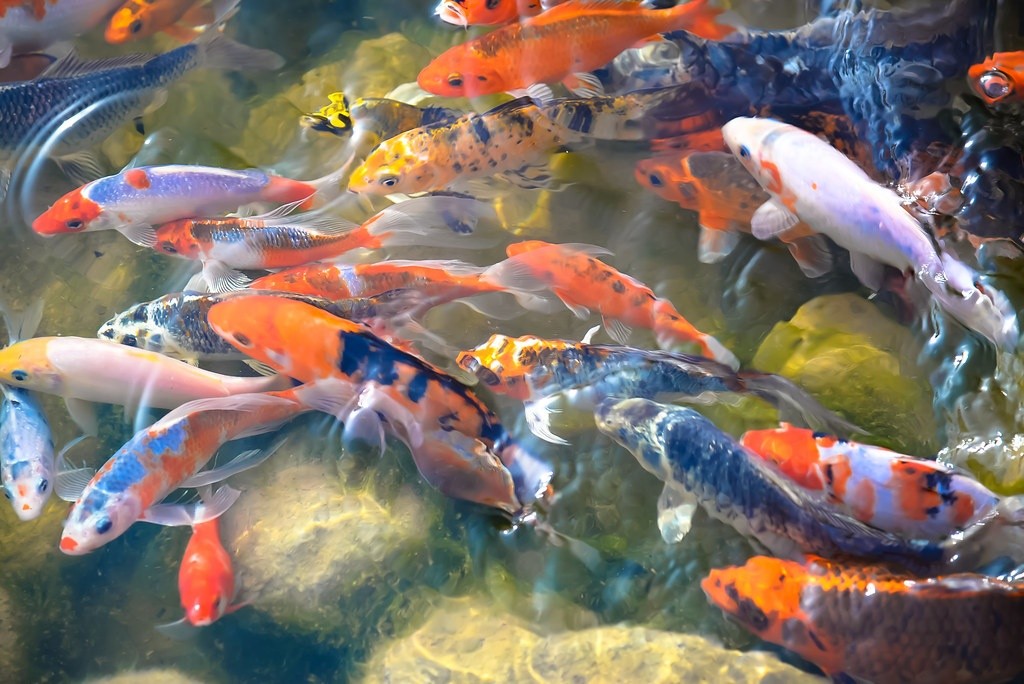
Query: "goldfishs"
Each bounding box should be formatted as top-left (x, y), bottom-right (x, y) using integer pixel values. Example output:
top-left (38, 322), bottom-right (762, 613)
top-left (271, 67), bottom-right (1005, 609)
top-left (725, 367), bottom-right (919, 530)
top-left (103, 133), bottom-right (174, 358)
top-left (0, 315), bottom-right (544, 626)
top-left (304, 0), bottom-right (1024, 355)
top-left (0, 0), bottom-right (306, 315)
top-left (455, 335), bottom-right (1024, 684)
